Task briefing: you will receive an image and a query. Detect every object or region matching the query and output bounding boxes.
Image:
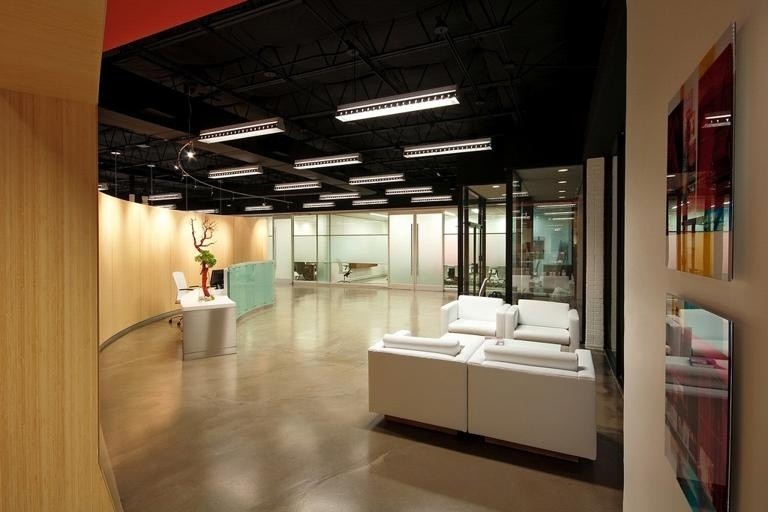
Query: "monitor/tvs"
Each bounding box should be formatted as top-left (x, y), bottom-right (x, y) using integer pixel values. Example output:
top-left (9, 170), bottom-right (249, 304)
top-left (210, 269), bottom-right (224, 289)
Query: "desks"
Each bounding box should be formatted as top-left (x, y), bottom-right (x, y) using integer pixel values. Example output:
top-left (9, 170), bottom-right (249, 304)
top-left (306, 261), bottom-right (346, 282)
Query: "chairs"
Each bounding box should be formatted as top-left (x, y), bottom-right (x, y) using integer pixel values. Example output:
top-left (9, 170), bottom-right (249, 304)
top-left (169, 272), bottom-right (200, 325)
top-left (367, 294), bottom-right (597, 463)
top-left (665, 307), bottom-right (730, 486)
top-left (294, 262), bottom-right (308, 280)
